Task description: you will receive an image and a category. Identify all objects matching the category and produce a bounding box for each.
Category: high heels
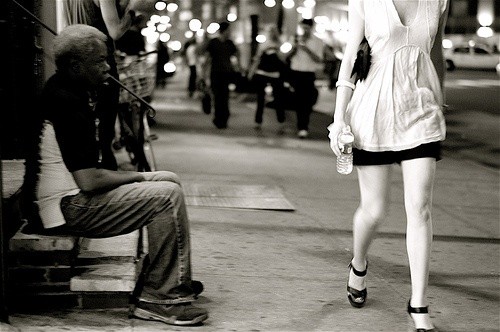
[347,257,368,308]
[408,299,435,332]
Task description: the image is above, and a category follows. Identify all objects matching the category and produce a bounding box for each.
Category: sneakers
[132,280,203,300]
[133,300,207,325]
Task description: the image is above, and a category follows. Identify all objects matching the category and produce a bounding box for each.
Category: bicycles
[116,51,158,173]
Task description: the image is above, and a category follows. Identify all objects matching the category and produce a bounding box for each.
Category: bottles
[337,125,354,175]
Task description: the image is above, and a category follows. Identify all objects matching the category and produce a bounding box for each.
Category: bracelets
[336,80,355,90]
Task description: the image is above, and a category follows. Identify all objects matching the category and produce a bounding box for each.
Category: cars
[443,42,500,72]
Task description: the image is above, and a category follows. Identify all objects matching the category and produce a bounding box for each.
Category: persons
[181,19,242,130]
[20,23,210,325]
[247,19,335,138]
[113,10,151,139]
[63,0,139,171]
[328,0,450,332]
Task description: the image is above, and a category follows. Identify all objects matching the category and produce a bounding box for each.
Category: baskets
[118,58,156,104]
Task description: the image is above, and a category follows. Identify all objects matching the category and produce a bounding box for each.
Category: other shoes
[254,122,263,132]
[278,123,286,133]
[301,131,308,138]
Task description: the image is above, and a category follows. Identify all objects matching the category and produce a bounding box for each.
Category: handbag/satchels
[202,93,211,113]
[350,37,371,81]
